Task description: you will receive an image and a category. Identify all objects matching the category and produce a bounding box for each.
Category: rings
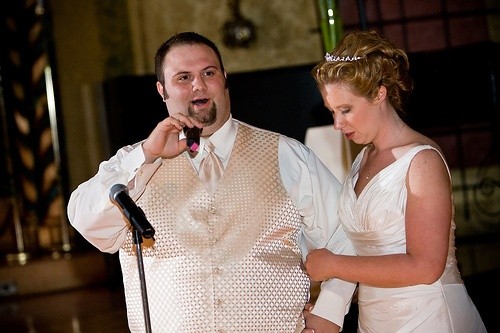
[309,306,314,311]
[312,329,315,333]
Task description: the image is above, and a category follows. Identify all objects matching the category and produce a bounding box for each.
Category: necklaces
[366,123,405,179]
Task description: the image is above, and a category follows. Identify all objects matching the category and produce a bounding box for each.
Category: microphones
[182,105,202,155]
[109,184,156,239]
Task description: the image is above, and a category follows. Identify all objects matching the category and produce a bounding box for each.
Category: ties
[196,139,227,197]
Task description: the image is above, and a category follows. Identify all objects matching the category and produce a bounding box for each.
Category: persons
[67,31,359,333]
[303,30,487,333]
